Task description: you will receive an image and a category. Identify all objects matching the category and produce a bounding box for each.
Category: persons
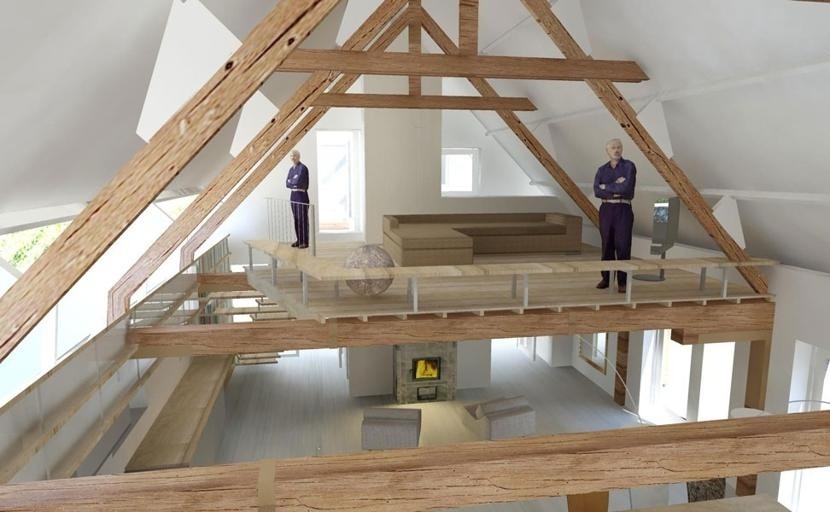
[285,148,310,249]
[592,138,637,293]
